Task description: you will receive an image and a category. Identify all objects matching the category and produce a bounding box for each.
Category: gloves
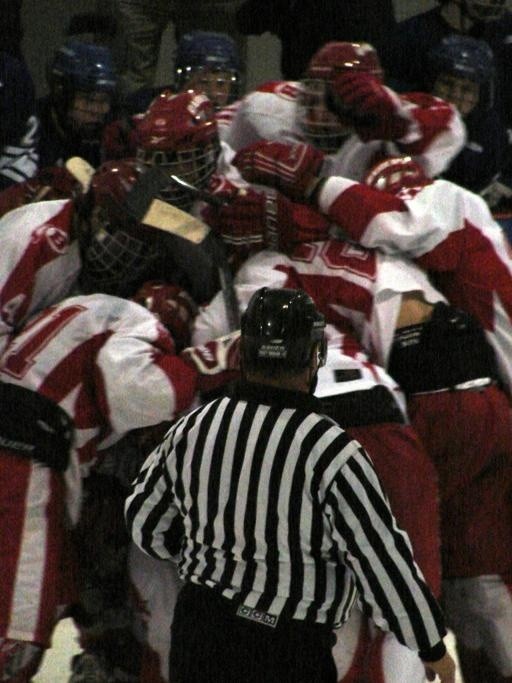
[182,330,244,394]
[321,70,417,145]
[201,186,332,254]
[229,135,327,202]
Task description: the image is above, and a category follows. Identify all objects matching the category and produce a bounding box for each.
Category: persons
[4,38,512,682]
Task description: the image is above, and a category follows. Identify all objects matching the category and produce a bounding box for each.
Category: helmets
[79,157,165,286]
[422,30,498,132]
[361,154,432,198]
[127,276,199,349]
[292,40,386,157]
[172,29,245,112]
[240,287,327,368]
[128,91,223,211]
[39,40,120,155]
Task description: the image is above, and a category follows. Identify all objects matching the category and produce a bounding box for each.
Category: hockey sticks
[169,172,222,243]
[138,198,240,332]
